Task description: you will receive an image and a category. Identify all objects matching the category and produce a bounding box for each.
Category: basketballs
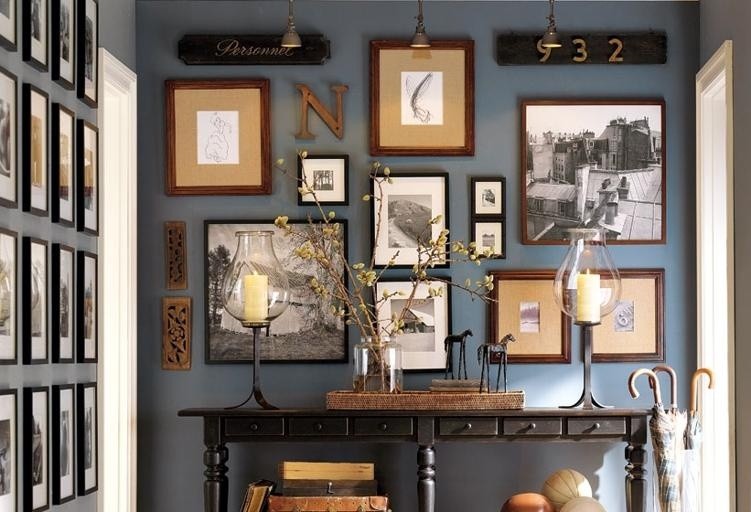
[542,468,593,511]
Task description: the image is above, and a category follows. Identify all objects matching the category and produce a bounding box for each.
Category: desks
[177,407,669,512]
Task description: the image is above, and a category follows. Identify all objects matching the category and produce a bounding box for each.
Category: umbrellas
[684,368,715,512]
[629,368,681,512]
[649,365,688,512]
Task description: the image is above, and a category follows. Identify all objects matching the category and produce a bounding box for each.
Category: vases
[352,336,404,393]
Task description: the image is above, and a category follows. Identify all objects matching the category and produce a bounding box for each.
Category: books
[240,461,378,512]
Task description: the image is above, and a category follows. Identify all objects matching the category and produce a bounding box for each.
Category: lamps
[409,1,431,47]
[541,0,563,48]
[282,0,304,48]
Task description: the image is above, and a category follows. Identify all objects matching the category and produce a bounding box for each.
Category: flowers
[270,147,500,394]
